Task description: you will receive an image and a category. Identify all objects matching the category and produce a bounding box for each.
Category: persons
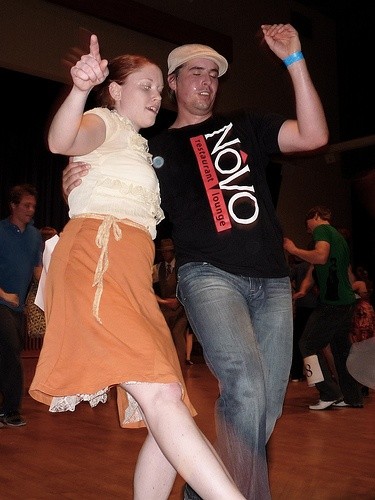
[44,33,250,500]
[0,180,374,426]
[61,22,328,500]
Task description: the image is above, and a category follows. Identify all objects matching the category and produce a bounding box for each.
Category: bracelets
[283,50,304,66]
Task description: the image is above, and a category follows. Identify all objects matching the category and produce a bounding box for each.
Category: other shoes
[309,397,343,410]
[333,401,362,407]
[185,360,194,366]
[0,414,27,428]
[184,483,203,500]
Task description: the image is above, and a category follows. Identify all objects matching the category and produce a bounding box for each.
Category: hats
[167,44,228,78]
[157,239,174,250]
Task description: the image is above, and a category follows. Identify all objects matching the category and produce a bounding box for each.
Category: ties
[167,265,171,279]
